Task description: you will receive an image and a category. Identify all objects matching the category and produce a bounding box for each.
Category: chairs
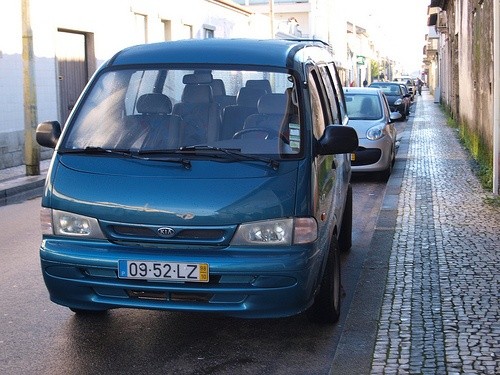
[112,93,183,149]
[173,85,222,147]
[221,88,268,140]
[391,87,396,91]
[360,97,373,113]
[240,93,287,139]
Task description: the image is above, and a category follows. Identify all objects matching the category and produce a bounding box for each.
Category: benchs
[198,80,272,109]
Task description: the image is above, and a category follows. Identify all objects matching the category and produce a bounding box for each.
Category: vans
[36,38,359,326]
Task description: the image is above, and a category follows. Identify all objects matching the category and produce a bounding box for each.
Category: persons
[417,81,423,95]
[363,79,367,87]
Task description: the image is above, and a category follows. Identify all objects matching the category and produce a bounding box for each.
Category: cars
[341,87,403,183]
[368,82,411,122]
[390,77,420,116]
